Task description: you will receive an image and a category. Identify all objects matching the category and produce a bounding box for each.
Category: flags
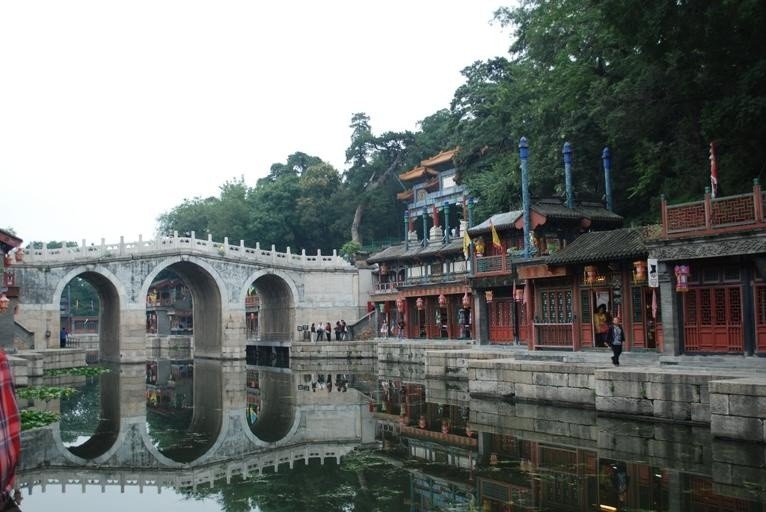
[491,224,501,249]
[709,142,718,193]
[463,229,471,261]
[652,288,657,319]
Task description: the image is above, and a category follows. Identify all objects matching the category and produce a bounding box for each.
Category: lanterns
[4,254,12,267]
[399,412,472,438]
[15,247,24,262]
[396,298,403,313]
[634,264,646,281]
[437,294,447,309]
[416,298,424,311]
[0,294,10,312]
[516,289,524,303]
[674,264,690,292]
[461,290,470,309]
[485,291,493,304]
[586,270,596,284]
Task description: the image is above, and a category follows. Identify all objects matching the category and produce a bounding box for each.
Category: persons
[381,379,407,400]
[605,317,625,367]
[448,224,453,237]
[397,316,405,339]
[381,319,389,339]
[60,328,68,348]
[611,462,629,504]
[311,320,348,343]
[594,304,611,348]
[312,374,348,392]
